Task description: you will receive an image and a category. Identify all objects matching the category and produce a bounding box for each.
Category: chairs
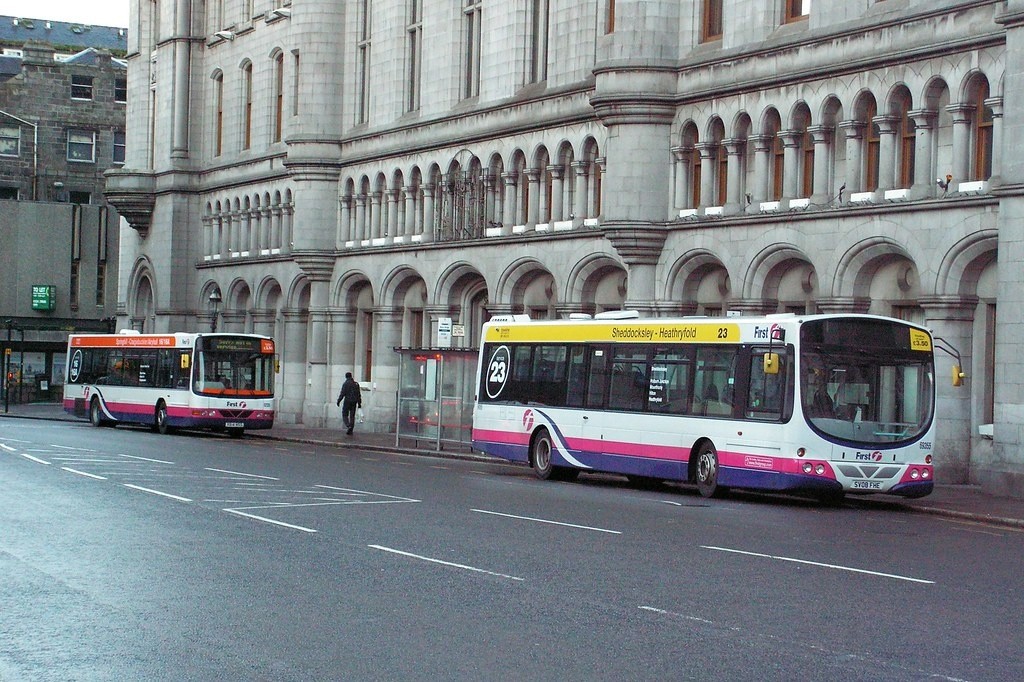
[814,390,838,419]
[695,384,734,417]
[514,358,643,403]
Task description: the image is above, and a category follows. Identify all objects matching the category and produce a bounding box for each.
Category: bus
[471,310,968,498]
[63,329,281,435]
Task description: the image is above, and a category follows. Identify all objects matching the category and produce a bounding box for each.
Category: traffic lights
[8,372,13,382]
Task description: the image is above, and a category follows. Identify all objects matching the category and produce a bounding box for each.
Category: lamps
[744,192,751,204]
[838,183,846,201]
[937,174,952,194]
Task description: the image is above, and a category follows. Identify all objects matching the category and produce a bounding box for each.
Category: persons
[337,372,362,435]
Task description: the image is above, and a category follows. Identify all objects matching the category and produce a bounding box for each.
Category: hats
[345,372,352,378]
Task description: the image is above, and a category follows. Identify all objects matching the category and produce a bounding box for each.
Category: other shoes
[346,426,353,435]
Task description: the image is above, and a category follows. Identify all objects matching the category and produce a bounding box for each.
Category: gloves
[337,399,340,407]
[358,404,362,408]
